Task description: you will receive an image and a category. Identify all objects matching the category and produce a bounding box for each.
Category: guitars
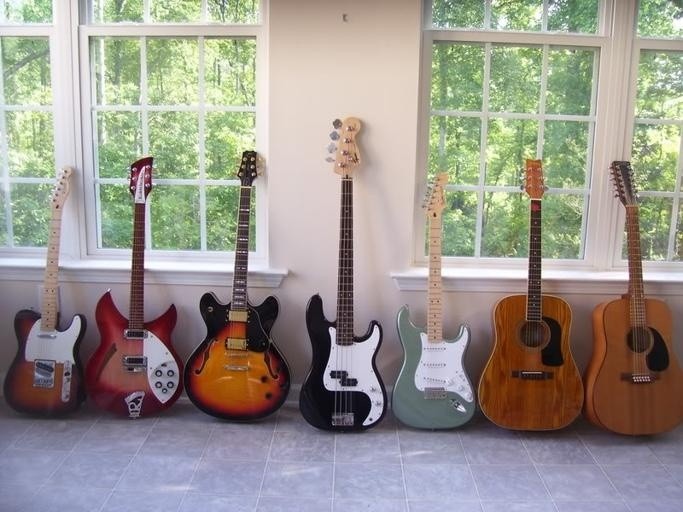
[4,167,87,418]
[391,170,477,431]
[299,118,387,432]
[478,159,585,432]
[86,158,184,419]
[183,150,292,422]
[583,161,683,437]
